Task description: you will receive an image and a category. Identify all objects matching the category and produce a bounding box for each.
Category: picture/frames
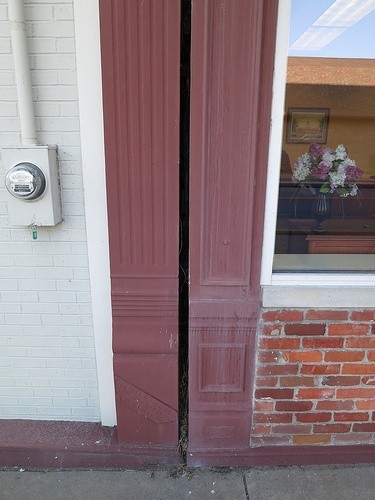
[285,106,331,145]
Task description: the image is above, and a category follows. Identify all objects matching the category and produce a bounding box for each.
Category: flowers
[289,143,365,198]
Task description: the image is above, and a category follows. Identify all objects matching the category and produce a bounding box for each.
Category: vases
[311,193,331,233]
